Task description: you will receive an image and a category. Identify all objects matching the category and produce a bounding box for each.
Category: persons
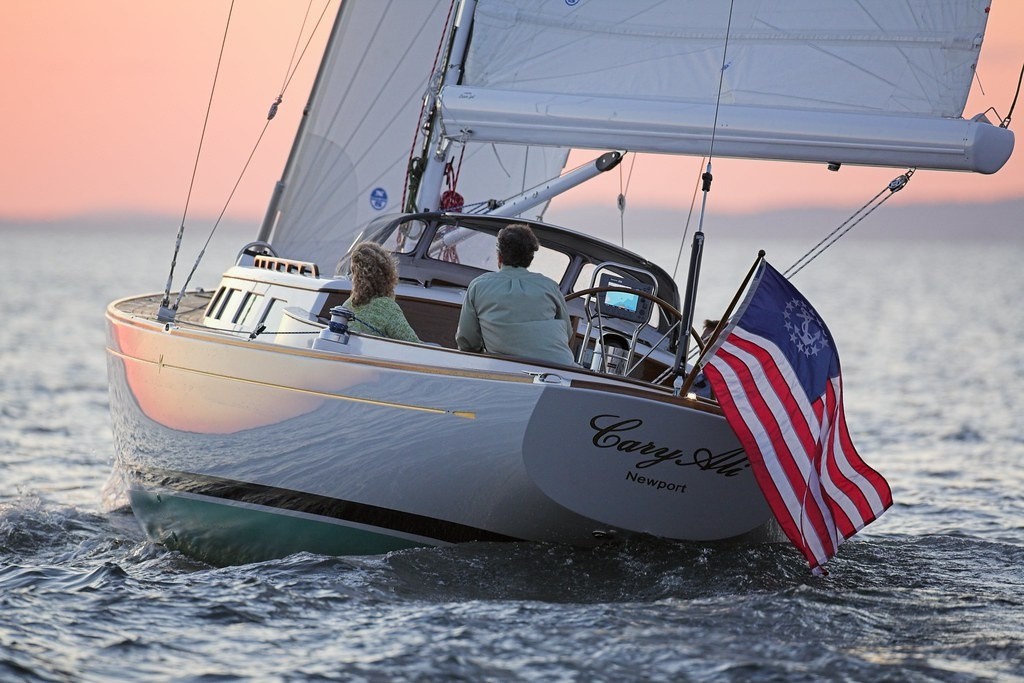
[456,224,577,366]
[342,242,423,347]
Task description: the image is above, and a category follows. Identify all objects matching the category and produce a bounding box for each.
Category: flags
[699,257,894,568]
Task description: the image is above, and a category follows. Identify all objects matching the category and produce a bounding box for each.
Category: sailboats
[106,0,1024,568]
